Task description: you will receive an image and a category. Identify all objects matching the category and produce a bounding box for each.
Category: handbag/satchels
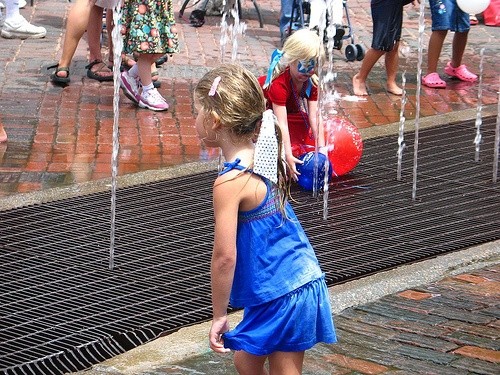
[482,0,500,27]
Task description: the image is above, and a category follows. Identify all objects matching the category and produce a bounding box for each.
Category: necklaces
[290,79,315,139]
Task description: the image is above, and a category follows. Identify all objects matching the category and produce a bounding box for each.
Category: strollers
[280,0,366,62]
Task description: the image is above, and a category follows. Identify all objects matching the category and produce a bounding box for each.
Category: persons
[195,0,352,49]
[0,0,48,40]
[422,1,478,88]
[0,121,8,149]
[193,68,337,375]
[352,0,414,97]
[47,0,181,111]
[251,28,329,182]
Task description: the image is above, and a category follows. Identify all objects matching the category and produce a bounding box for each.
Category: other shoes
[134,52,167,66]
[334,26,345,41]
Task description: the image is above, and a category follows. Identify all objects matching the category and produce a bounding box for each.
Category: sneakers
[1,15,46,39]
[121,71,138,103]
[0,0,27,8]
[139,88,169,110]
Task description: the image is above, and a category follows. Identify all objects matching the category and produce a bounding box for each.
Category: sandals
[421,72,446,88]
[152,71,161,87]
[85,59,113,81]
[47,64,70,83]
[108,56,132,72]
[445,62,478,82]
